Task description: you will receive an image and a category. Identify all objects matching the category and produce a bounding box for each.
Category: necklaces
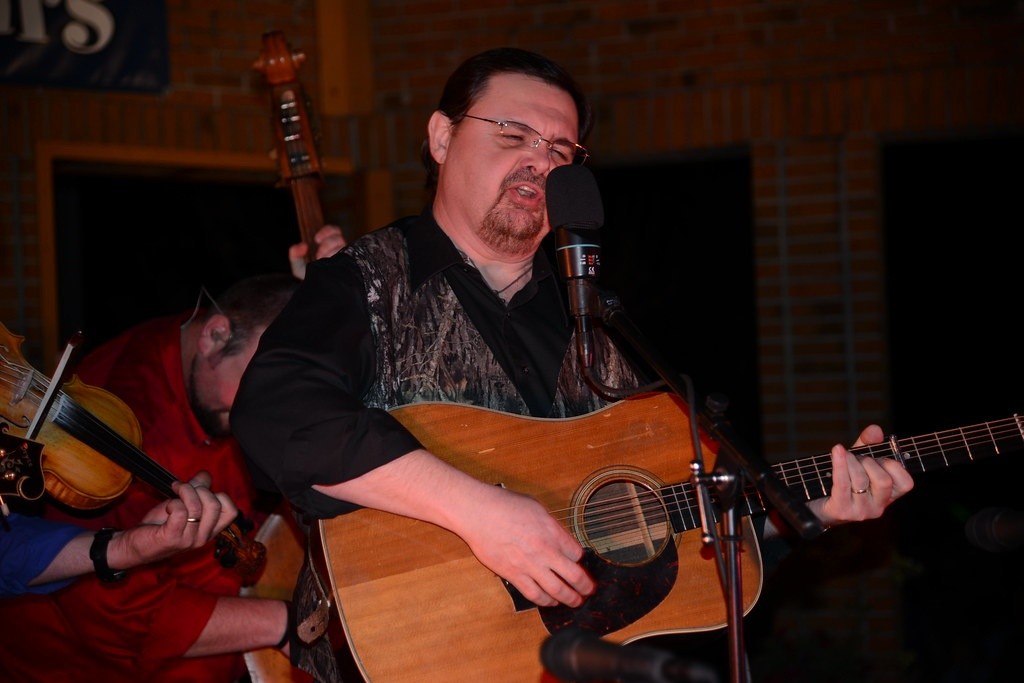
[459,250,532,304]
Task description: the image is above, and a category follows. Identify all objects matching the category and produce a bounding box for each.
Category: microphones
[541,627,719,683]
[965,507,1024,553]
[545,163,604,372]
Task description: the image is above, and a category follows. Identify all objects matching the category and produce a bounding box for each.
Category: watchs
[89,527,128,583]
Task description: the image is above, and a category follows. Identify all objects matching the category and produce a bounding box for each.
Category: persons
[0,469,239,598]
[229,48,916,683]
[0,224,350,683]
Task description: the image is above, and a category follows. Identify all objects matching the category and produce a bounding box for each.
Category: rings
[852,485,871,494]
[188,518,201,523]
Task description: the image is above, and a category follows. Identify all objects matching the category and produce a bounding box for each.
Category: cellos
[231,25,362,683]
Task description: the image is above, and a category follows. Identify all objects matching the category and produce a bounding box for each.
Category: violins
[0,320,268,569]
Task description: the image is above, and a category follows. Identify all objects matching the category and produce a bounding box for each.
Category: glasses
[462,113,595,168]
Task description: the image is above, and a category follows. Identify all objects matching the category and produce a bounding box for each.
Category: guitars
[307,375,1024,683]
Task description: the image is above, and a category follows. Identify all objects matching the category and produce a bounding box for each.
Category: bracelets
[275,599,294,650]
